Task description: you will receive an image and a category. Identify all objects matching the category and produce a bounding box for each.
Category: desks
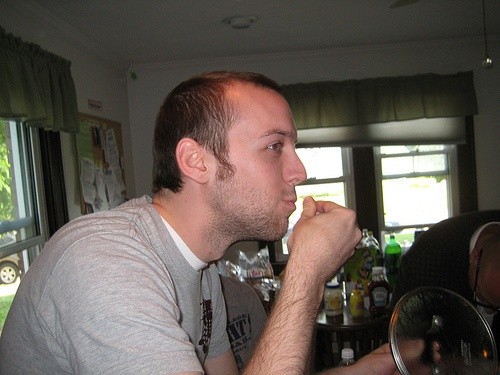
[315,300,389,369]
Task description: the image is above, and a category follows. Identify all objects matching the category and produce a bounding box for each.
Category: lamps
[222,15,258,29]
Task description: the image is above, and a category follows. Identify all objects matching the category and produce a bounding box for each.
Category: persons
[0,71,440,375]
[393,208,500,366]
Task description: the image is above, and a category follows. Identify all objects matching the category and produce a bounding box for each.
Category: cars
[0,255,20,285]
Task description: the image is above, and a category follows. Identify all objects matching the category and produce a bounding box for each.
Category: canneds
[350,287,364,318]
[324,282,344,317]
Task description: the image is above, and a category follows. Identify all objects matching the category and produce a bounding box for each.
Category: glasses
[471,248,500,312]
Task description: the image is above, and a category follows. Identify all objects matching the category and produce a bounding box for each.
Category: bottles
[344,227,402,318]
[338,347,355,368]
[324,282,344,318]
